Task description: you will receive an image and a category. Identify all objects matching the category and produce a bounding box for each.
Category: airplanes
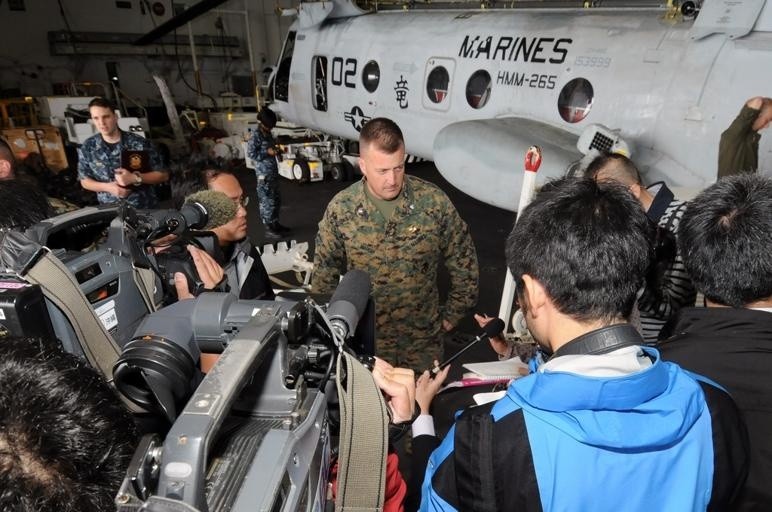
[255,1,771,215]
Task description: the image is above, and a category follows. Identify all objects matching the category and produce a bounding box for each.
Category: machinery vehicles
[0,95,71,182]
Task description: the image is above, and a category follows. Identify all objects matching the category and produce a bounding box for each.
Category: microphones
[427,318,506,376]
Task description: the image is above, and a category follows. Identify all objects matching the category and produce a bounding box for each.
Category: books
[462,356,528,381]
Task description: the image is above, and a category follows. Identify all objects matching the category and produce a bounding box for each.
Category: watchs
[134,172,142,183]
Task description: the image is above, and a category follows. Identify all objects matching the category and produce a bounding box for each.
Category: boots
[265,221,292,238]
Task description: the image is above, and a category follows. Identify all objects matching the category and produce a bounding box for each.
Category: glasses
[233,195,250,208]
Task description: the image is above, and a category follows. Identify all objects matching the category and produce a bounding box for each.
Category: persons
[418,178,752,512]
[585,152,690,348]
[655,174,772,512]
[77,97,171,208]
[1,179,225,373]
[416,359,451,479]
[0,334,416,512]
[246,107,289,236]
[0,139,17,181]
[165,156,276,302]
[312,118,479,376]
[473,312,552,393]
